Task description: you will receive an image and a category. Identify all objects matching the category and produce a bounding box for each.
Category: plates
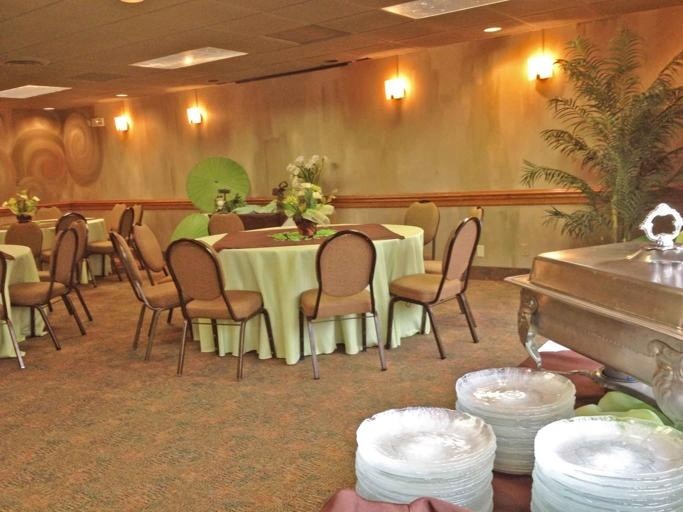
[527,416,681,511]
[453,369,576,476]
[352,405,495,512]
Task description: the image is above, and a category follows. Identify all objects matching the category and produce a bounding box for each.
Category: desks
[324,339,608,512]
[502,240,682,426]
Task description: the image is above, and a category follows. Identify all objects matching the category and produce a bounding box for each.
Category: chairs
[404,202,441,260]
[297,228,386,380]
[1,211,97,370]
[132,222,175,325]
[208,213,247,233]
[108,230,193,365]
[87,203,144,287]
[385,206,482,360]
[166,239,277,380]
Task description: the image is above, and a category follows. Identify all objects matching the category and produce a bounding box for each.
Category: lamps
[114,116,130,133]
[384,77,404,100]
[527,54,553,80]
[187,107,202,124]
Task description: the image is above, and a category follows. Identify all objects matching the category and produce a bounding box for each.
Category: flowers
[281,154,337,227]
[2,185,39,217]
